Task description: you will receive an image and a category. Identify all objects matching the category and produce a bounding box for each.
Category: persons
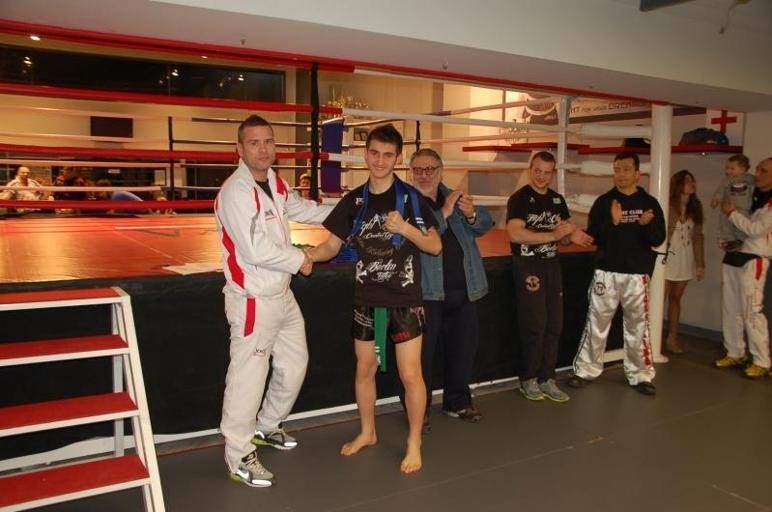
[60,166,89,215]
[395,144,499,438]
[206,110,338,492]
[565,149,668,398]
[92,178,151,214]
[0,164,48,217]
[710,152,756,253]
[660,168,708,356]
[303,121,446,477]
[43,172,65,197]
[294,171,312,200]
[711,156,772,381]
[503,149,597,405]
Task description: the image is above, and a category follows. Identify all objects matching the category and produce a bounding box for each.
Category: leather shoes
[669,340,685,356]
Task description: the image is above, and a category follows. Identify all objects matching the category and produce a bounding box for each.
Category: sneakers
[725,241,744,252]
[520,377,544,400]
[636,383,655,397]
[253,424,297,450]
[746,363,769,378]
[228,454,276,488]
[541,378,570,403]
[570,374,592,386]
[403,409,432,434]
[444,408,485,423]
[714,354,746,367]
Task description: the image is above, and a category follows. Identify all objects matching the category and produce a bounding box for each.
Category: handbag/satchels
[679,128,728,145]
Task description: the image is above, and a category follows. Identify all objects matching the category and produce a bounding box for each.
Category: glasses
[412,167,440,176]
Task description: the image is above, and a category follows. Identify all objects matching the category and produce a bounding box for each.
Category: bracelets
[465,211,478,220]
